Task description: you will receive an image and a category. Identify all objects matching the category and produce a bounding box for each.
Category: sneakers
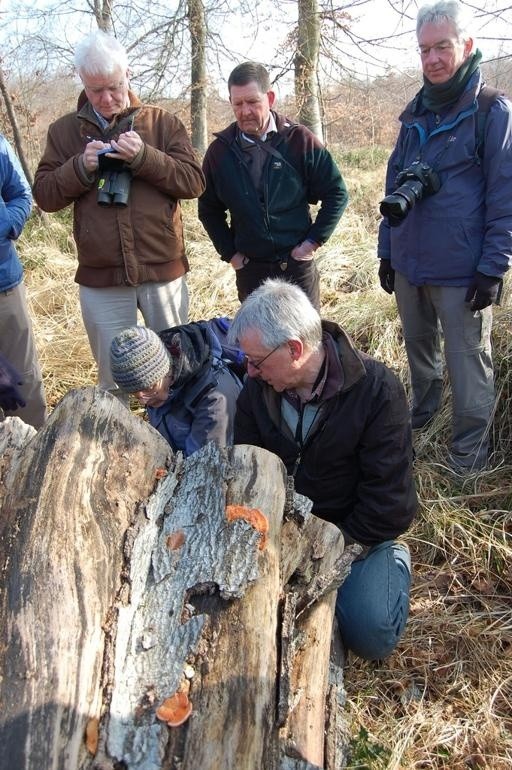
[393,540,411,569]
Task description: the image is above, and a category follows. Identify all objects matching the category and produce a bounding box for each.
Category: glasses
[241,347,279,371]
[416,44,455,56]
[131,385,162,401]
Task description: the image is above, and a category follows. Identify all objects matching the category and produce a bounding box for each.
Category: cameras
[379,162,441,227]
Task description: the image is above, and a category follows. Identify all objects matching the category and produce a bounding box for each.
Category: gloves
[377,260,396,294]
[0,360,26,415]
[465,271,501,312]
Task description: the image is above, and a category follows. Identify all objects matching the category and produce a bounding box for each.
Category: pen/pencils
[86,136,97,142]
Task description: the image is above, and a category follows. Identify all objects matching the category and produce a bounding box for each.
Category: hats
[110,326,174,391]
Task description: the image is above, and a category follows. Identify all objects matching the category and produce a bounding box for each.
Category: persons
[0,354,26,413]
[197,62,349,318]
[376,0,512,475]
[232,276,418,661]
[110,316,243,459]
[31,29,207,413]
[0,128,48,428]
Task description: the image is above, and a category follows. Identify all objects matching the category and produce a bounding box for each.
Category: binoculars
[97,169,132,209]
